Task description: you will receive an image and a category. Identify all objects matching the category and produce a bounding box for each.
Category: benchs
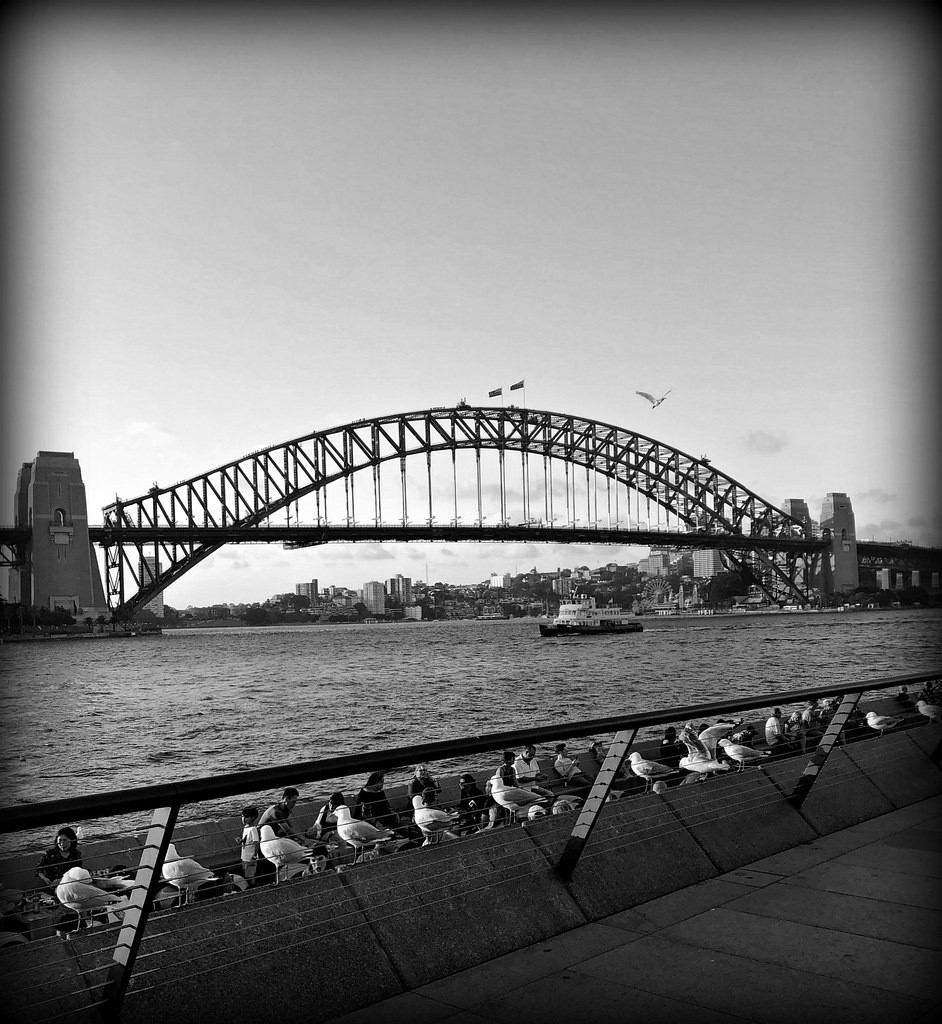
[0,692,929,951]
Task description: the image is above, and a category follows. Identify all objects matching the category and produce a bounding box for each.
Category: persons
[765,696,845,752]
[512,744,554,807]
[469,781,504,822]
[895,679,942,709]
[699,720,759,749]
[235,806,259,886]
[408,764,442,816]
[314,793,346,855]
[555,743,595,787]
[449,774,485,814]
[660,726,688,766]
[257,788,328,858]
[36,827,83,895]
[354,772,399,833]
[496,751,519,788]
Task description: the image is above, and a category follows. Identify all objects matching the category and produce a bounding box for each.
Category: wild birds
[625,752,679,794]
[260,825,313,887]
[57,867,122,934]
[162,844,218,907]
[329,805,394,865]
[914,700,942,726]
[636,390,669,410]
[717,737,771,773]
[863,712,904,738]
[412,795,460,846]
[487,775,548,826]
[679,722,735,780]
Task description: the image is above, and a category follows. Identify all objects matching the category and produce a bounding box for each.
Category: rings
[55,879,57,881]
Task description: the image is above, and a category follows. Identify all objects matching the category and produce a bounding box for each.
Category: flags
[510,380,523,391]
[488,388,502,398]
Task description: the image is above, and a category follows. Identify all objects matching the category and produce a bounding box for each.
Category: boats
[539,593,644,637]
[478,613,510,620]
